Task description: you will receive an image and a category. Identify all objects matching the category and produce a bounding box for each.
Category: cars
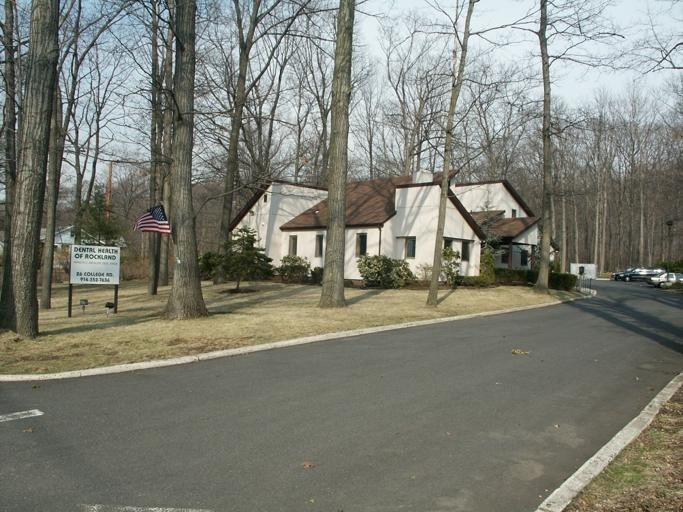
[609,266,665,282]
[646,271,682,289]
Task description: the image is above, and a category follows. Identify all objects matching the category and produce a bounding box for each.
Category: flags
[131,203,171,235]
